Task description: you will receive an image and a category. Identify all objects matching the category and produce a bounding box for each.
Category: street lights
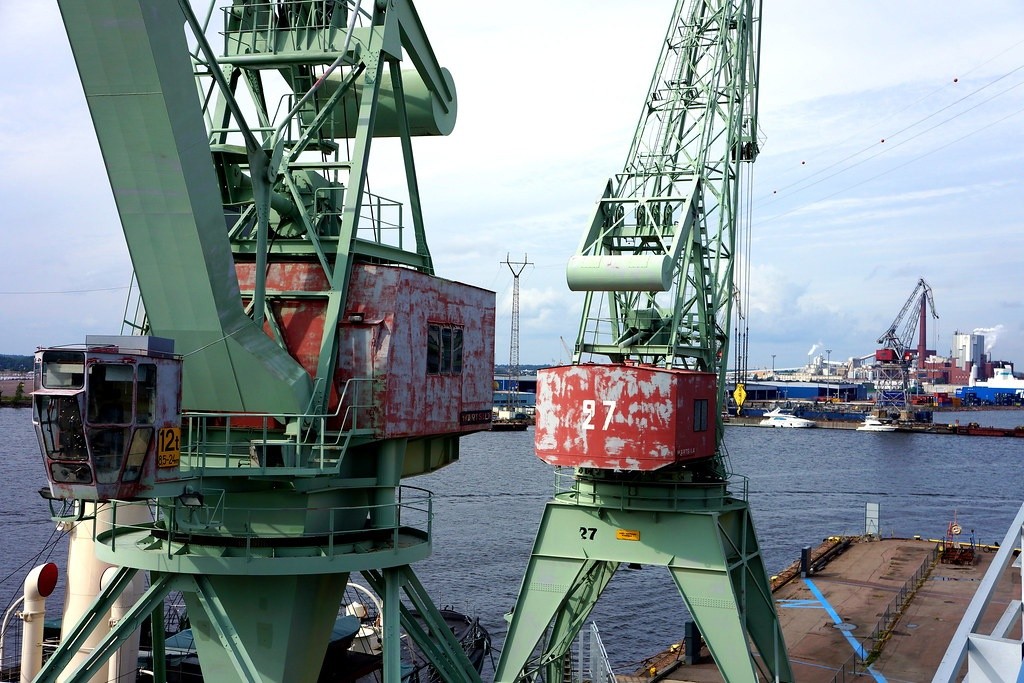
[783,390,788,403]
[825,349,832,402]
[776,390,781,402]
[771,354,777,376]
[834,393,838,398]
[844,392,849,402]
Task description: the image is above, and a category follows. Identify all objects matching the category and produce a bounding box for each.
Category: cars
[721,411,736,418]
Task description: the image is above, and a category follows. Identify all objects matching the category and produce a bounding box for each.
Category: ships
[605,498,1024,683]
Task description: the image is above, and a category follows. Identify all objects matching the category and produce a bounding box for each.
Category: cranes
[559,335,574,366]
[492,0,802,681]
[20,3,505,681]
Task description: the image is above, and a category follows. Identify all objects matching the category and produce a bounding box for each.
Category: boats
[856,415,900,431]
[490,407,528,432]
[0,576,491,683]
[759,403,817,429]
